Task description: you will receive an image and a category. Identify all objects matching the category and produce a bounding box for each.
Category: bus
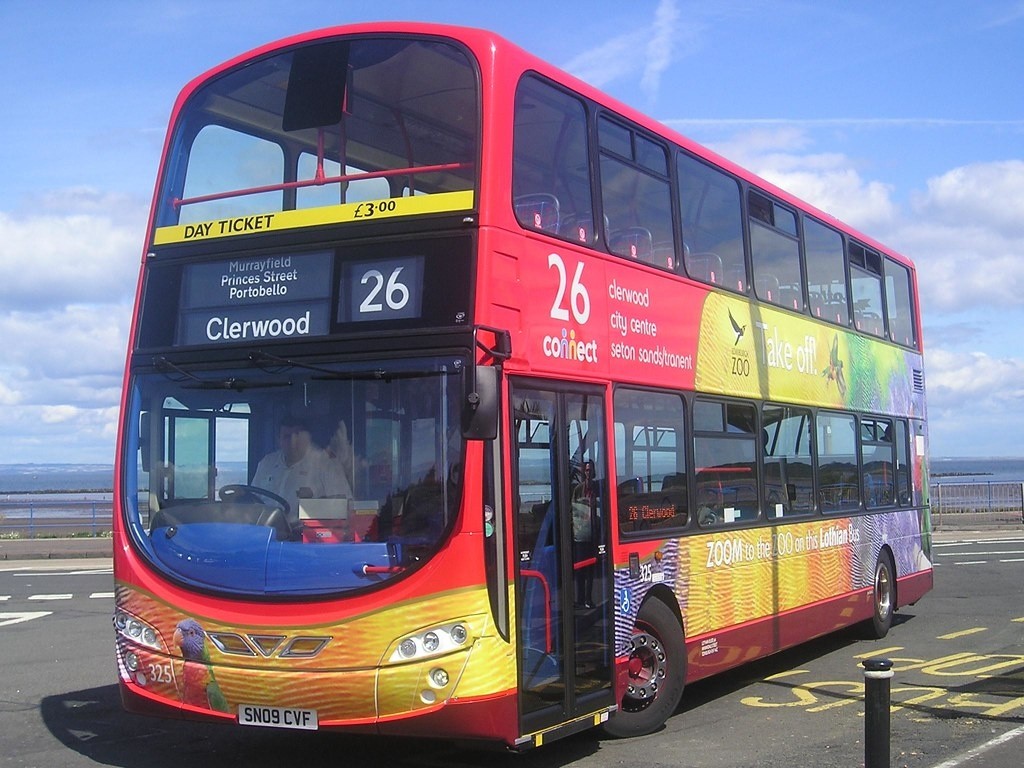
[111,21,934,751]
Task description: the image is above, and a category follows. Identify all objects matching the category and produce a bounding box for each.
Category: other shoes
[571,602,584,609]
[585,602,596,608]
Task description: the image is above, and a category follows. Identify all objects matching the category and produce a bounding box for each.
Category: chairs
[570,457,911,537]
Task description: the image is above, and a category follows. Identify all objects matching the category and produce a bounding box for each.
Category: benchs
[514,191,912,346]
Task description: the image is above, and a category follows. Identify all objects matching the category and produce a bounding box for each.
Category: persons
[251,417,354,531]
[573,461,596,611]
